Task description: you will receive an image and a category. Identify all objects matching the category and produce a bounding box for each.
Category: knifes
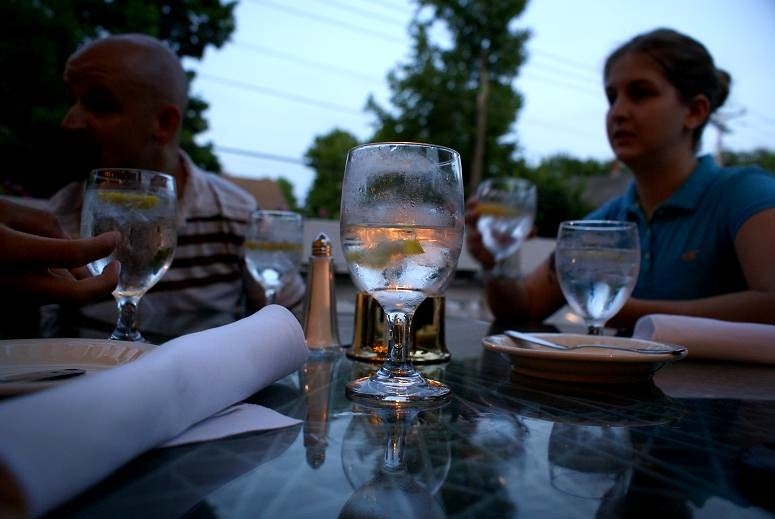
[503,327,686,354]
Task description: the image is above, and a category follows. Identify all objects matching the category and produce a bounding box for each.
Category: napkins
[160,402,304,447]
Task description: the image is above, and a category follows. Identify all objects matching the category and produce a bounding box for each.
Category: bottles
[301,231,343,350]
[297,356,336,467]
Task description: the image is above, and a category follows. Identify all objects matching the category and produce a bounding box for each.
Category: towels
[653,364,775,402]
[633,312,775,363]
[0,302,307,518]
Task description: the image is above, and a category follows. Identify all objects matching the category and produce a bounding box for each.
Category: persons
[465,29,775,328]
[0,34,306,344]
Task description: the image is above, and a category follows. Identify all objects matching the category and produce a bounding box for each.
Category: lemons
[98,191,158,209]
[475,204,518,215]
[348,239,422,261]
[245,241,303,249]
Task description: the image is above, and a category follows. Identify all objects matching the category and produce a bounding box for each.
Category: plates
[481,333,688,381]
[0,337,159,396]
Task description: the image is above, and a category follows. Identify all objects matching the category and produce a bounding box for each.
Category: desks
[0,309,775,519]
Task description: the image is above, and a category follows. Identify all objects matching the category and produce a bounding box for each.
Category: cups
[549,422,632,499]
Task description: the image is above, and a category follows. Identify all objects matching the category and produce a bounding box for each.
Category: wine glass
[555,219,641,336]
[474,176,537,282]
[81,168,177,344]
[339,141,465,404]
[338,397,452,519]
[244,209,304,307]
